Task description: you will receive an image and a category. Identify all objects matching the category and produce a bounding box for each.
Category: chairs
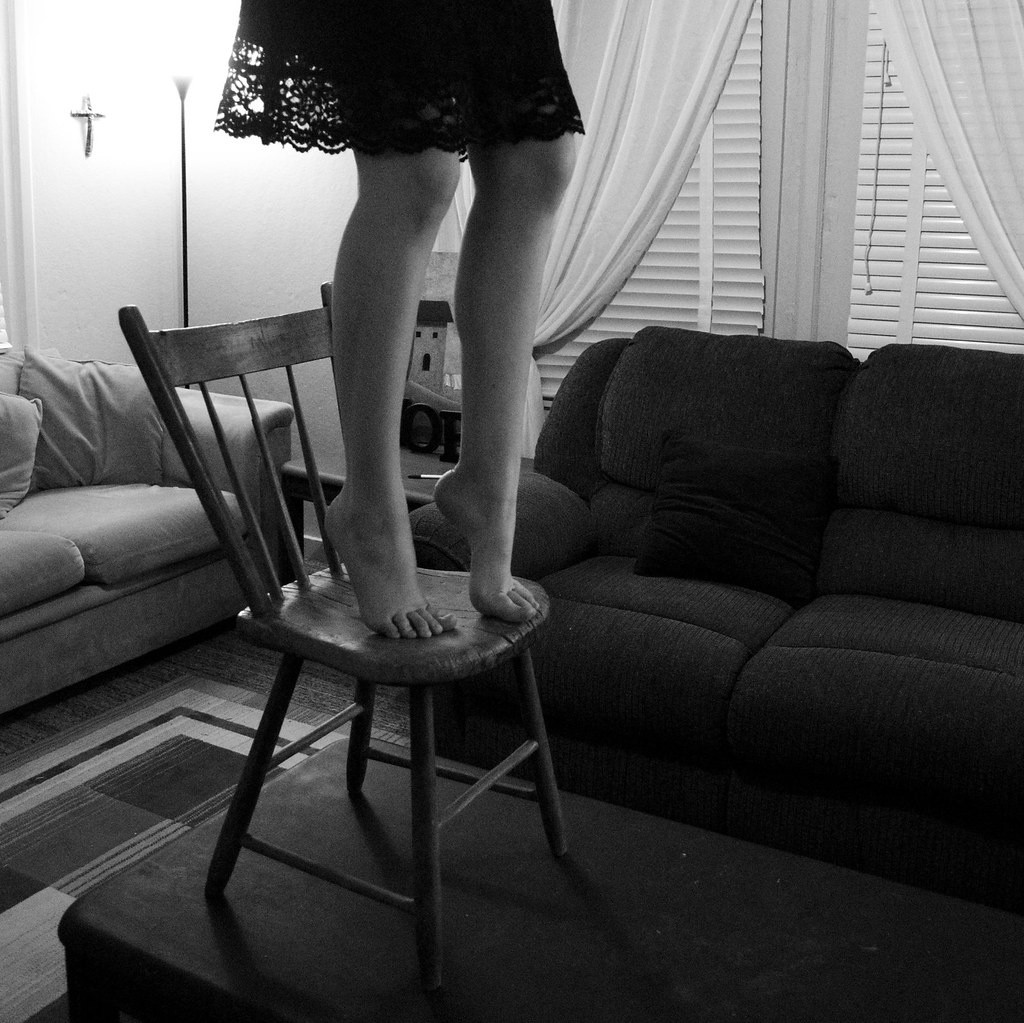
[119,280,568,992]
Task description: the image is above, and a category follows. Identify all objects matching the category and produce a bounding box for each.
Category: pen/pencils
[408,474,446,480]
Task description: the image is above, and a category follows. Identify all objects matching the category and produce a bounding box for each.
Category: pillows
[631,430,837,608]
[17,346,165,486]
[0,392,43,519]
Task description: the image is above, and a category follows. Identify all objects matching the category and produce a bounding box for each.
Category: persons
[214,0,586,636]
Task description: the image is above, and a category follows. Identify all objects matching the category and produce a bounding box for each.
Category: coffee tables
[57,738,1024,1023]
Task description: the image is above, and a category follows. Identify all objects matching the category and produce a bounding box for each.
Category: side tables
[277,446,533,586]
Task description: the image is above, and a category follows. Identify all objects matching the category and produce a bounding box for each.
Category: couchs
[406,326,1023,916]
[0,346,296,730]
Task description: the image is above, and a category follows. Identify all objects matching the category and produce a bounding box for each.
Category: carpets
[0,673,410,1023]
